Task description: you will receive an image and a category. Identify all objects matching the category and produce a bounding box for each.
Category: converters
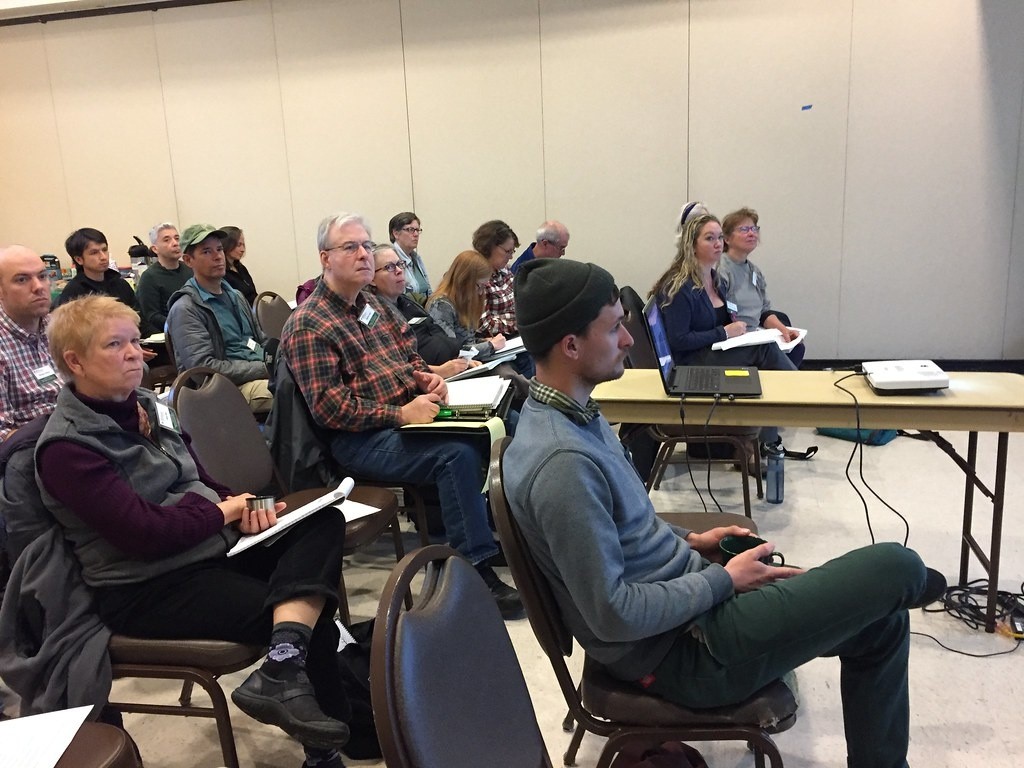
[1010,617,1024,641]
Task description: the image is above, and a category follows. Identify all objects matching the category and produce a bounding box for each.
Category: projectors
[860,358,951,396]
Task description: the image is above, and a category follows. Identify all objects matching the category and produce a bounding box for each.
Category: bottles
[138,263,148,284]
[62,264,77,278]
[764,441,785,504]
[121,272,134,278]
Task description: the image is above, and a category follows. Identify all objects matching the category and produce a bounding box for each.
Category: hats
[512,258,613,349]
[179,223,228,253]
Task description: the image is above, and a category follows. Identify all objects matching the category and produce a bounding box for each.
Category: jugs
[128,236,158,279]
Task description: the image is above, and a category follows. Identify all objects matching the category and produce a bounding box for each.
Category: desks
[590,369,1024,633]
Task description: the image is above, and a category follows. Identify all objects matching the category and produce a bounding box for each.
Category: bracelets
[724,327,728,339]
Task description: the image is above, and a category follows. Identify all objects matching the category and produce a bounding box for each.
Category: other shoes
[914,567,947,610]
[489,578,527,620]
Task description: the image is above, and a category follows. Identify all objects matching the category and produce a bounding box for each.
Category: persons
[0,243,73,446]
[500,257,946,768]
[282,212,569,613]
[651,208,805,459]
[32,294,382,767]
[60,222,276,421]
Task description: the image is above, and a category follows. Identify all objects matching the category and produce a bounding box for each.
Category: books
[482,336,526,361]
[437,379,504,408]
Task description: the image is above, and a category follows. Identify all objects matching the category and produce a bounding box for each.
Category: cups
[245,496,276,518]
[720,535,785,567]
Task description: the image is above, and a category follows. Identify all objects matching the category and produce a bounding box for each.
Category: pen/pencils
[413,394,448,408]
[487,328,494,338]
[731,314,736,322]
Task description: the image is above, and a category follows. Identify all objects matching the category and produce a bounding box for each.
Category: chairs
[37,286,799,768]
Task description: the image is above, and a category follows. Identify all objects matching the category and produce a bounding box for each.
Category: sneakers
[232,669,351,749]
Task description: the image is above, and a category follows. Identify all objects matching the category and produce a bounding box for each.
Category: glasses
[732,226,760,233]
[495,244,516,256]
[401,227,422,234]
[375,260,406,272]
[542,239,568,251]
[325,240,377,254]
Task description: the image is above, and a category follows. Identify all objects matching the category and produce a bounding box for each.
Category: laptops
[642,295,763,398]
